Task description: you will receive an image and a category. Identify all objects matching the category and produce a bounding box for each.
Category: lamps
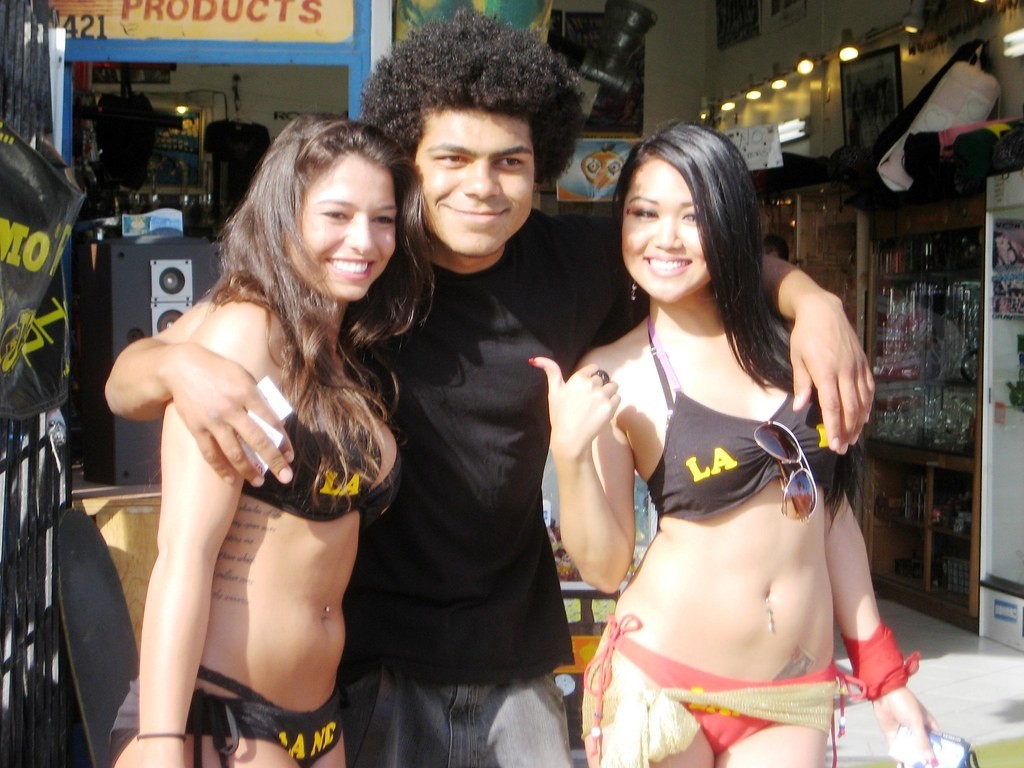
[697,0,925,120]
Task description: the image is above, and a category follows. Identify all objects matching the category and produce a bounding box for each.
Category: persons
[106,4,877,768]
[529,120,941,768]
[763,235,788,262]
[108,114,433,768]
[993,232,1024,313]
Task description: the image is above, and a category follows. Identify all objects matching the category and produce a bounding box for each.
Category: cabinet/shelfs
[755,185,866,334]
[862,193,993,639]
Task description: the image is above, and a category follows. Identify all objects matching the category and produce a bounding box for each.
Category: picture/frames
[838,44,903,158]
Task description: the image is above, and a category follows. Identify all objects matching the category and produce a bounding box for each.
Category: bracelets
[137,733,187,742]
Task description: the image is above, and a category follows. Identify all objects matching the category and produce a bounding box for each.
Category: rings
[591,369,609,386]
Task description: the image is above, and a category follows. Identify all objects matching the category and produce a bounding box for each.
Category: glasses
[754,420,817,523]
